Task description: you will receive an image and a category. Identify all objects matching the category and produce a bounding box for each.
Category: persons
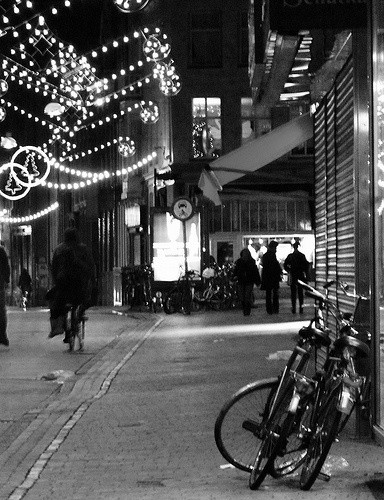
[230,249,262,316]
[45,226,97,343]
[0,244,12,347]
[192,105,215,158]
[18,267,34,302]
[283,241,310,316]
[202,252,231,270]
[260,241,283,315]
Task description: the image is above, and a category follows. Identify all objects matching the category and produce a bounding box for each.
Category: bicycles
[165,264,254,315]
[65,304,85,352]
[214,279,372,490]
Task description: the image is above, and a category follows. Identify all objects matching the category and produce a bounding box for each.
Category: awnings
[195,106,312,207]
